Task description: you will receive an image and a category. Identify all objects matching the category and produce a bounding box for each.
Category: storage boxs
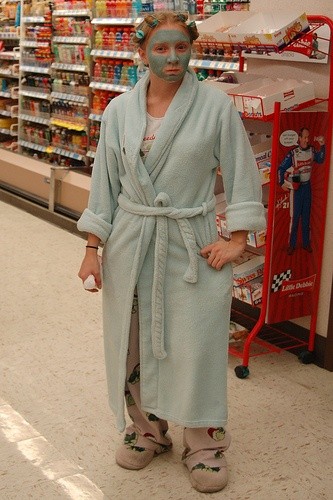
[190,9,318,380]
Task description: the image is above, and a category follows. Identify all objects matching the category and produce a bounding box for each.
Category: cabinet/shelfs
[1,0,252,249]
[181,15,333,378]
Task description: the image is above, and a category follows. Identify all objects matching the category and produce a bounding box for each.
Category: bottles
[0,0,248,175]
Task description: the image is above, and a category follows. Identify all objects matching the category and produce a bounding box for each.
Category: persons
[78,10,267,493]
[277,127,326,255]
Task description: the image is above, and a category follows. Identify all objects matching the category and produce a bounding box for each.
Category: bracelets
[86,246,98,250]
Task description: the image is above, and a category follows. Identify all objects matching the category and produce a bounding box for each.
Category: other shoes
[116,444,154,470]
[287,247,294,255]
[189,466,228,493]
[303,246,312,253]
